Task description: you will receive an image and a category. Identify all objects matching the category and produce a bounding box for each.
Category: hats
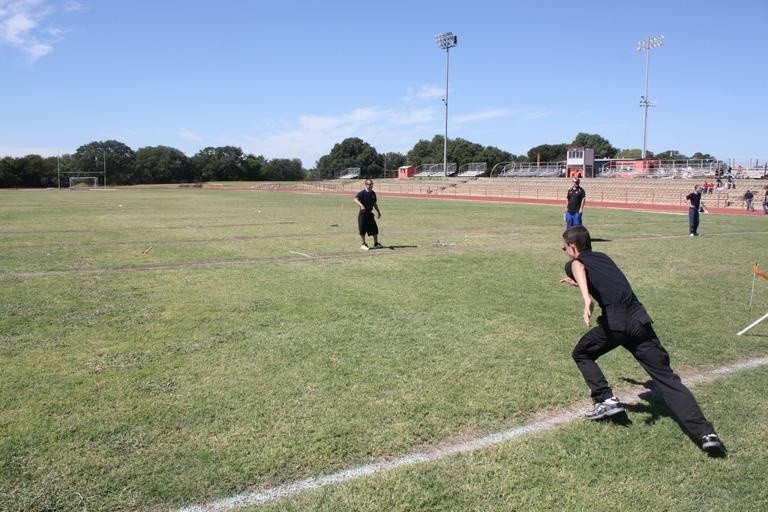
[573,178,580,183]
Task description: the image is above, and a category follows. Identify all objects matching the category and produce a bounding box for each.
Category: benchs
[296,173,767,214]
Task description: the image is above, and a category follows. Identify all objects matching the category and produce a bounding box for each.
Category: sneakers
[361,243,382,250]
[701,433,727,457]
[585,397,625,420]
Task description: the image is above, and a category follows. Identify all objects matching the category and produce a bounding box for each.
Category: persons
[714,176,721,188]
[561,178,586,251]
[706,182,713,193]
[557,227,728,456]
[702,181,707,193]
[353,179,381,249]
[726,174,735,188]
[762,185,767,213]
[685,183,703,236]
[743,190,755,212]
